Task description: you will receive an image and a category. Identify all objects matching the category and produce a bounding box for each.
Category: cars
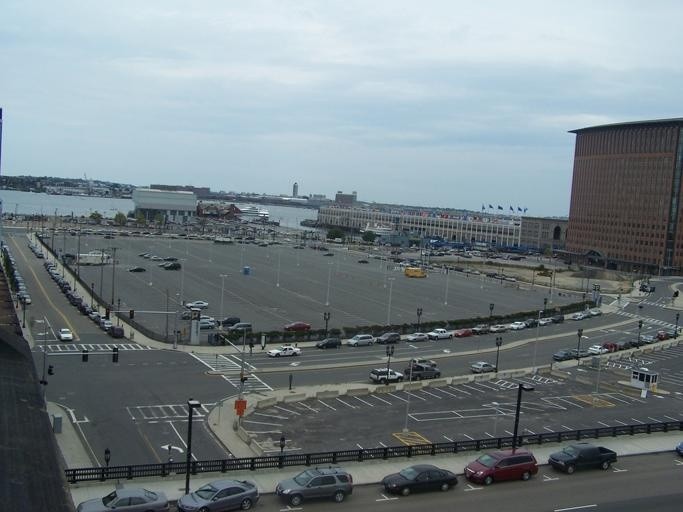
[178,298,314,331]
[175,232,281,246]
[41,260,127,341]
[54,248,62,252]
[266,344,302,358]
[376,244,543,286]
[568,306,604,322]
[1,237,31,308]
[314,310,564,350]
[549,327,675,364]
[359,259,368,265]
[128,251,183,273]
[27,241,46,260]
[640,282,657,293]
[44,224,166,241]
[470,360,498,374]
[677,438,683,458]
[283,237,334,258]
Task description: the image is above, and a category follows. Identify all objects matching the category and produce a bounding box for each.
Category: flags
[481,204,527,213]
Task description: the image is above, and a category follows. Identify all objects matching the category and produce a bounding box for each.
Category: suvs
[369,357,442,382]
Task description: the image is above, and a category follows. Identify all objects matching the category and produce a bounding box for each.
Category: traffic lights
[47,364,55,379]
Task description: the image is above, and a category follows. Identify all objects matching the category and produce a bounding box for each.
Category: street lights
[413,305,425,332]
[673,311,681,339]
[509,381,534,455]
[490,333,504,371]
[636,319,644,349]
[542,296,549,316]
[183,395,207,496]
[380,340,395,379]
[487,301,496,327]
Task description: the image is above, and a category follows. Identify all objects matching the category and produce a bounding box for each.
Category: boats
[240,205,282,226]
[300,218,322,228]
